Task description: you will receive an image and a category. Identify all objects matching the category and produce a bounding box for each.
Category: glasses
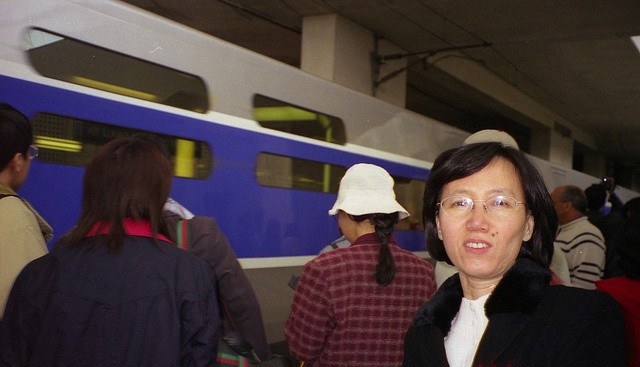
[27,145,40,161]
[437,195,526,218]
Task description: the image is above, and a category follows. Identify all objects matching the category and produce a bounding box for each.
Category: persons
[584,184,627,280]
[401,140,633,367]
[549,184,608,292]
[594,195,640,367]
[158,195,269,365]
[1,102,55,320]
[601,175,625,213]
[1,134,223,366]
[435,129,574,292]
[282,163,437,367]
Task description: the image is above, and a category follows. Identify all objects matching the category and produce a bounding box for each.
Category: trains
[0,0,640,346]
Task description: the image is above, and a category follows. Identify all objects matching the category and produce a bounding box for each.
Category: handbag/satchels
[215,337,305,367]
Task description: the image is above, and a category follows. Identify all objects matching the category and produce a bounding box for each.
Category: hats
[328,163,410,221]
[463,129,520,150]
